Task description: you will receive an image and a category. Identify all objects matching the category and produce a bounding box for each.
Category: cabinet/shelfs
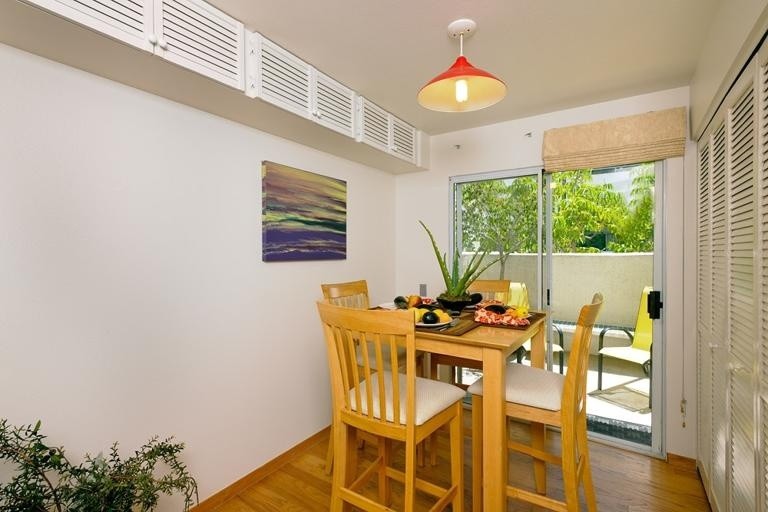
[689,45,767,512]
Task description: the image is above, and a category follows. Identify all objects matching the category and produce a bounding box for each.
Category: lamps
[416,20,508,115]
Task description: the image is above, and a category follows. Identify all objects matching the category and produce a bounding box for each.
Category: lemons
[408,307,451,323]
[518,308,528,318]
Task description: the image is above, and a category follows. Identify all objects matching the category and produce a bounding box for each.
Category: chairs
[318,281,651,512]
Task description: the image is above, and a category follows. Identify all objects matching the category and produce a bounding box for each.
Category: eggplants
[416,304,460,316]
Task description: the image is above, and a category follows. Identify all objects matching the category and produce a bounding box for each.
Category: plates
[416,320,452,327]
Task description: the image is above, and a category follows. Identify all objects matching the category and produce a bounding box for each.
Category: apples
[409,296,422,308]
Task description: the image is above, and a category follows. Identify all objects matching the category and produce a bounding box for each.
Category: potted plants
[419,220,517,314]
